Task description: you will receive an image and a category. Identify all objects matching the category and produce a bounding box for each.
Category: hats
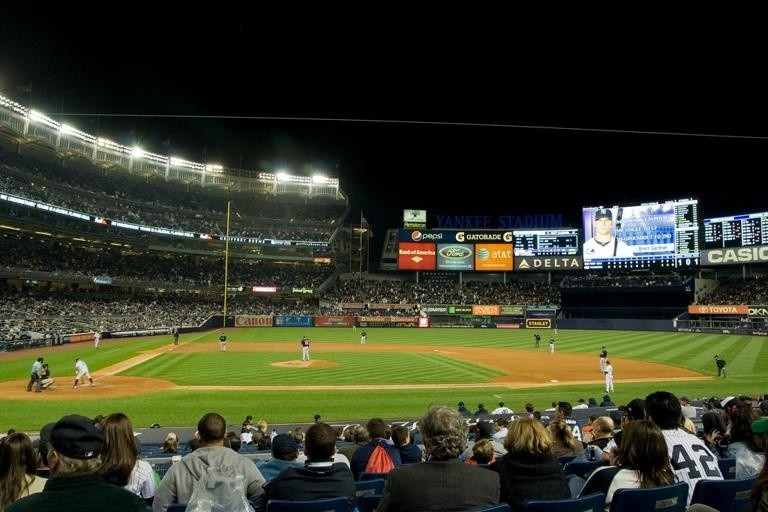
[719,395,743,410]
[617,397,647,421]
[49,414,104,460]
[272,433,302,453]
[474,420,494,438]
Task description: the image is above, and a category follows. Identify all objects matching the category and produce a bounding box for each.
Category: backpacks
[362,444,398,475]
[181,453,255,512]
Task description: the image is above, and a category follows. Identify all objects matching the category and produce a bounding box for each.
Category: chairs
[137,419,767,511]
